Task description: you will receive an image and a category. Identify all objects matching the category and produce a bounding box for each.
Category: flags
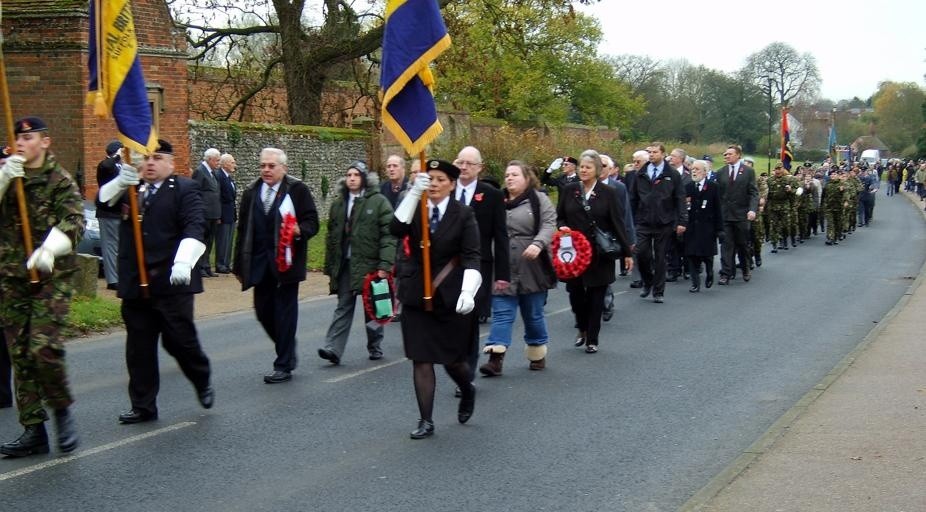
[828,119,837,154]
[85,0,162,156]
[378,0,451,159]
[781,106,793,175]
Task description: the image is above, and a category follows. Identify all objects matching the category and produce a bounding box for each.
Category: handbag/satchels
[593,226,624,260]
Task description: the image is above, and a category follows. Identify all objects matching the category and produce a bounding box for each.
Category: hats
[155,139,173,155]
[563,156,578,164]
[760,162,811,176]
[106,141,123,155]
[480,175,502,189]
[744,156,753,167]
[0,144,12,157]
[702,155,712,162]
[15,117,49,135]
[829,162,868,175]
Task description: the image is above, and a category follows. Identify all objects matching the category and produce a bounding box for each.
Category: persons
[233,147,319,383]
[318,160,398,365]
[886,157,926,200]
[94,139,215,423]
[0,147,12,409]
[541,145,767,353]
[0,117,87,457]
[383,147,559,440]
[216,155,238,273]
[95,141,126,290]
[759,157,883,253]
[191,148,221,277]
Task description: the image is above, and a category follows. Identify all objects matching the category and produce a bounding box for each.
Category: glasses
[602,164,609,168]
[456,159,481,167]
[633,159,647,164]
[258,161,285,170]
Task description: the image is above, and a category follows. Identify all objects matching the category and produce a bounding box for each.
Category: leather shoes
[196,376,214,408]
[603,292,614,321]
[618,269,664,303]
[119,407,157,422]
[665,258,762,292]
[410,418,435,439]
[200,269,237,277]
[318,348,340,364]
[264,339,297,383]
[574,332,599,353]
[369,351,382,359]
[455,385,475,424]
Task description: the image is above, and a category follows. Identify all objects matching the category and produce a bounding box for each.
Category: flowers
[402,234,411,258]
[474,192,484,202]
[548,229,592,279]
[274,210,297,274]
[362,272,401,325]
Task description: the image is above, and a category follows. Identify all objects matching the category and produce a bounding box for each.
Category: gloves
[0,155,28,199]
[26,227,74,274]
[455,268,483,316]
[100,162,141,208]
[169,238,208,287]
[393,171,431,225]
[546,157,563,174]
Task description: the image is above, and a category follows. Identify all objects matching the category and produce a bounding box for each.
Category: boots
[479,344,508,376]
[524,344,547,370]
[1,421,49,457]
[766,224,825,252]
[825,217,872,245]
[54,408,79,452]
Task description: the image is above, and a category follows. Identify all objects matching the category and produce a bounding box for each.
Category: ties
[460,189,465,203]
[652,168,657,182]
[696,183,700,191]
[730,166,734,182]
[264,188,274,215]
[429,208,440,234]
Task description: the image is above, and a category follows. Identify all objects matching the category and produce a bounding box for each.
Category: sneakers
[106,282,117,289]
